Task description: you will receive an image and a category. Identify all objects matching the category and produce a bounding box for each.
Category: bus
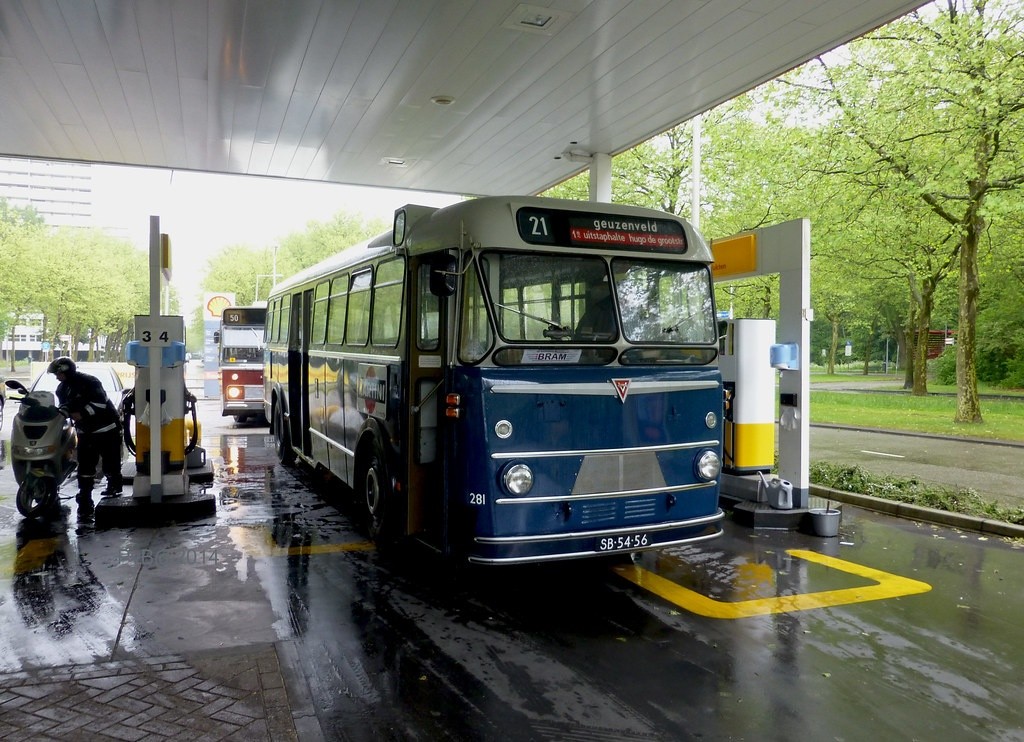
[213,302,270,423]
[263,195,725,569]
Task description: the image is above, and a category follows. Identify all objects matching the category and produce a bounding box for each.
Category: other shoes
[76,489,92,501]
[101,486,122,495]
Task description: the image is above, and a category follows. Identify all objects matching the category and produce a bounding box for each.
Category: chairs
[577,293,648,343]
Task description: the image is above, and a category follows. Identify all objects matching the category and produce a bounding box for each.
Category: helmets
[47,355,76,373]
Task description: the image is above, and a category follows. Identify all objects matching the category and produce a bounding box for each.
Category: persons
[46,356,124,507]
[571,284,652,360]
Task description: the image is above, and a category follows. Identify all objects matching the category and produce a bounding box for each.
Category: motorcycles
[6,380,79,519]
